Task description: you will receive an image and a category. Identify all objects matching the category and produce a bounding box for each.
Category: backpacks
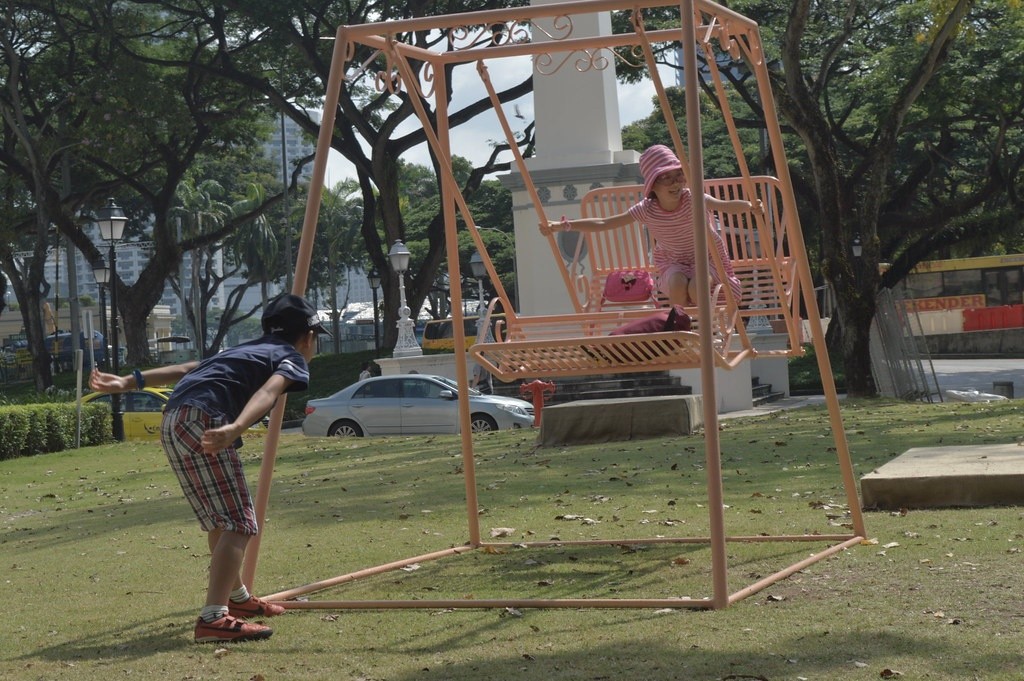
[602,269,654,301]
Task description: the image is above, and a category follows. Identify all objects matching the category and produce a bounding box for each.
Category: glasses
[655,173,684,185]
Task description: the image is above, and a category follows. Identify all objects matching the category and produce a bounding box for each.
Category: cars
[301,374,536,437]
[70,387,270,443]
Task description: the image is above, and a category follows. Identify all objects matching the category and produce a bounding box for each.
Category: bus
[878,254,1024,359]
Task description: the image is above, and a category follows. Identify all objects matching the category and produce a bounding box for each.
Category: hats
[261,294,333,337]
[639,144,682,197]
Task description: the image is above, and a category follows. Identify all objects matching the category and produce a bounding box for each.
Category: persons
[89,292,334,641]
[538,144,768,306]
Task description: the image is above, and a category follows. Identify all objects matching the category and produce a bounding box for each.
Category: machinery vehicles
[9,301,106,375]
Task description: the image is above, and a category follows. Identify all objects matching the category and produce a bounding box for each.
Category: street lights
[386,239,423,358]
[367,264,382,358]
[92,254,113,374]
[475,226,518,314]
[468,250,499,358]
[93,197,130,443]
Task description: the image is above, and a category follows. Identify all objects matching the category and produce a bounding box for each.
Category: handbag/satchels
[590,303,692,363]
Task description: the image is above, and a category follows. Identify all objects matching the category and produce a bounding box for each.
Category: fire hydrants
[518,379,554,428]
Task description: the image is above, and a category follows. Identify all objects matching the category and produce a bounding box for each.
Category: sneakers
[193,613,273,643]
[228,594,286,618]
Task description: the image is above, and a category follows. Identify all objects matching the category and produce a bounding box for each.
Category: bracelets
[561,214,571,233]
[130,368,147,391]
